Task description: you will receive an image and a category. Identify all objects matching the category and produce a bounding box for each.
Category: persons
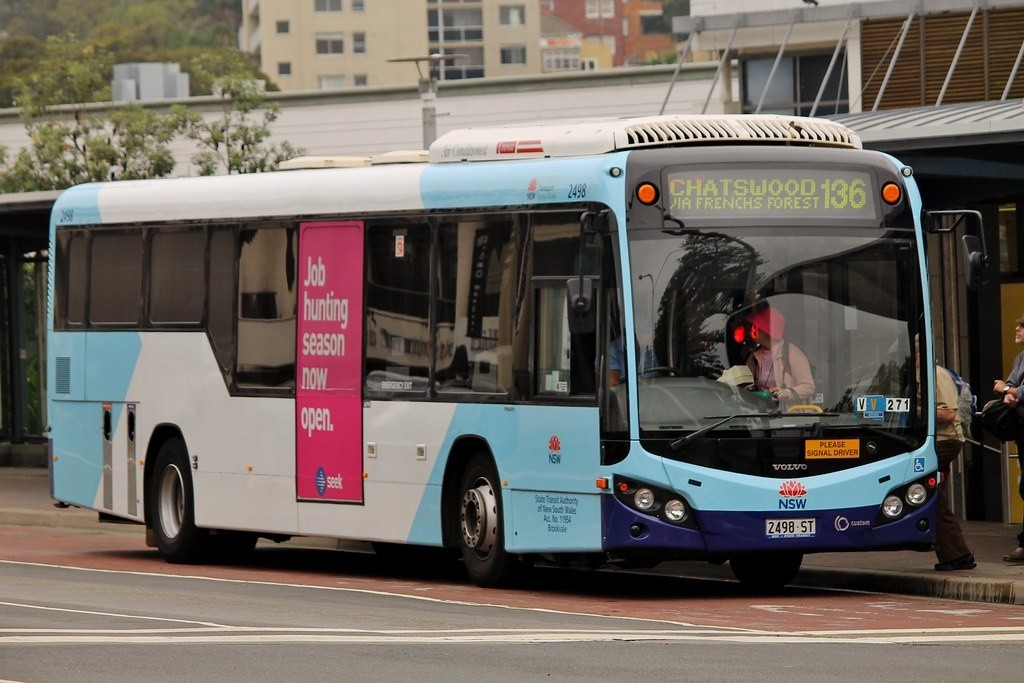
[993,315,1024,564]
[607,316,660,387]
[745,305,816,401]
[918,334,977,572]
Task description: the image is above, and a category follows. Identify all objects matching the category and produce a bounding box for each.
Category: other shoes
[934,552,976,570]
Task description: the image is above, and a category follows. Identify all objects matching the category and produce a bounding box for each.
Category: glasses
[1016,323,1024,328]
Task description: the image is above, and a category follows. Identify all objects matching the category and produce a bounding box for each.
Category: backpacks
[948,369,978,426]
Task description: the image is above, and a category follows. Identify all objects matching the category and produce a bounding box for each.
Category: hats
[1015,312,1024,323]
[744,307,786,340]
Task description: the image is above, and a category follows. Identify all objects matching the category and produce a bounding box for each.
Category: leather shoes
[1004,547,1024,561]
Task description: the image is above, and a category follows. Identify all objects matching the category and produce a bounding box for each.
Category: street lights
[384,54,469,152]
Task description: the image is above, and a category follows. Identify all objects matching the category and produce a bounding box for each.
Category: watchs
[1003,385,1011,395]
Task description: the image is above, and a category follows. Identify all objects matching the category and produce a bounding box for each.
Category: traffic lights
[724,298,781,410]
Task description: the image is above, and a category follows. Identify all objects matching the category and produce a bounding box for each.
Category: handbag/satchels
[981,380,1024,443]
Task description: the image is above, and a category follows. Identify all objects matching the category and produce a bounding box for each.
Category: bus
[45,112,994,588]
